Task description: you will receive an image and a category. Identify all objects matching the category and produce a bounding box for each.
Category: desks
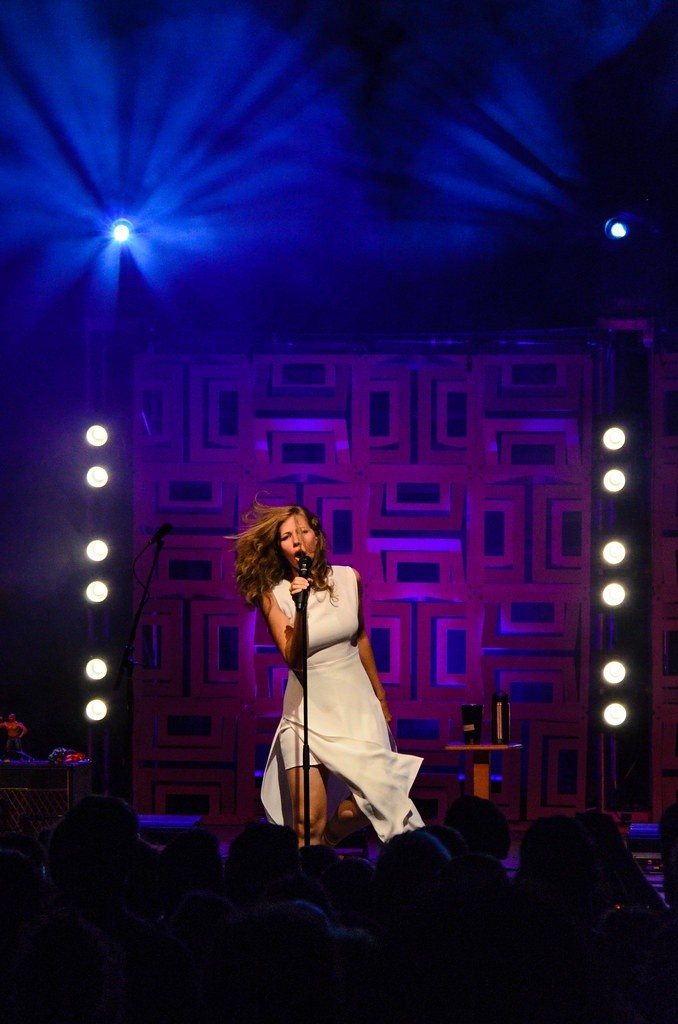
[446,743,523,801]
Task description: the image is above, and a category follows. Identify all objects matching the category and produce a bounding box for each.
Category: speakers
[0,759,93,840]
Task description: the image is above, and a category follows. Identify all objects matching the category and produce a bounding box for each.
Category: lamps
[592,649,648,733]
[598,532,639,611]
[593,416,641,499]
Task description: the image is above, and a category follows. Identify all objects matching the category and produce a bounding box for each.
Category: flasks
[491,690,511,745]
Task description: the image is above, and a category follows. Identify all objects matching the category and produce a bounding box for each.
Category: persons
[231,506,425,853]
[0,795,678,1024]
[0,714,27,752]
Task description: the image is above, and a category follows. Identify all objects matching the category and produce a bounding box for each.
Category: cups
[461,703,483,745]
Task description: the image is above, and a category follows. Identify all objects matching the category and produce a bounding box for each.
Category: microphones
[148,523,173,545]
[296,556,313,611]
[17,813,36,833]
